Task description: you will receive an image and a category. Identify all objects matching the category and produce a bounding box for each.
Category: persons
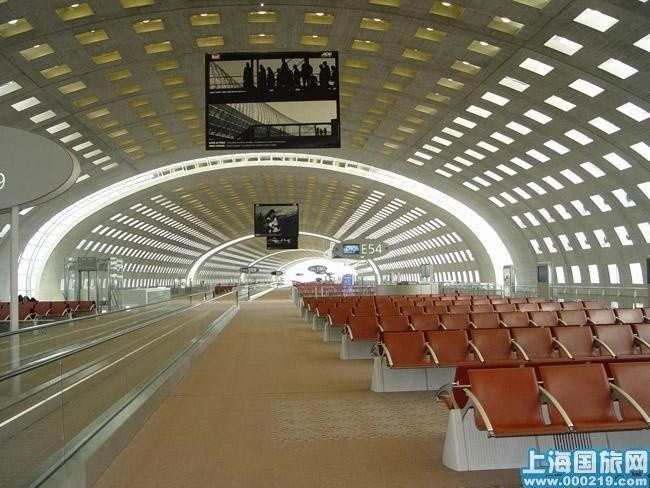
[30,298,35,302]
[243,57,338,90]
[18,295,23,302]
[316,128,327,136]
[23,296,29,302]
[263,209,281,234]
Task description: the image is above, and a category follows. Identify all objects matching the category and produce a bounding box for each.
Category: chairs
[213,285,236,293]
[289,278,649,471]
[0,299,97,325]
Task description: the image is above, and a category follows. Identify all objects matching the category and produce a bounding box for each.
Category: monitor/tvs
[343,244,360,254]
